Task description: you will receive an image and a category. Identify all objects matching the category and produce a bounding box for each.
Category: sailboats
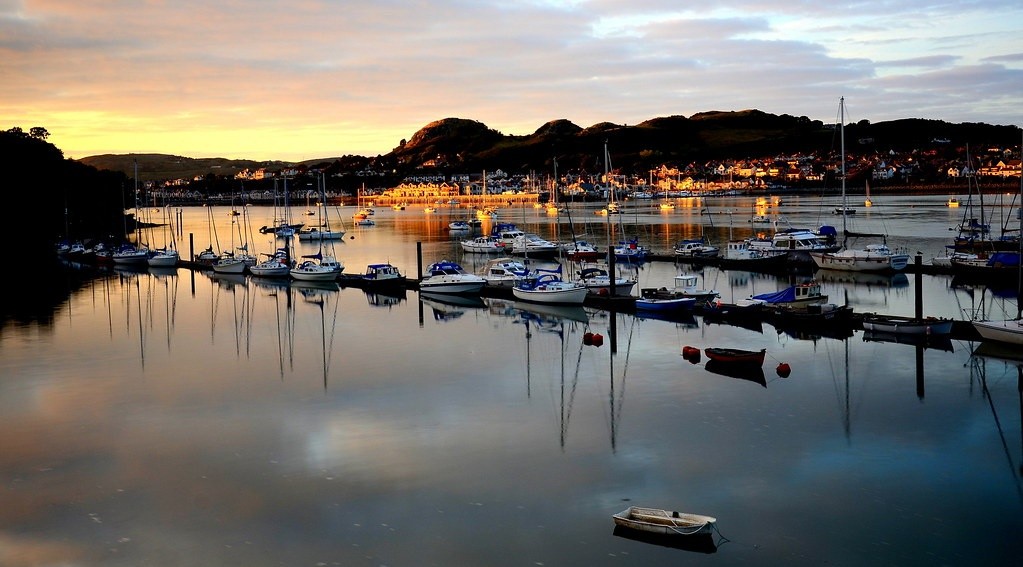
[809,95,910,271]
[932,143,1022,275]
[197,172,344,280]
[205,273,340,392]
[55,162,180,267]
[60,262,179,371]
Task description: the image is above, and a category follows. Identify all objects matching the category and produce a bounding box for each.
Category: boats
[863,331,955,354]
[705,348,766,365]
[361,285,407,312]
[261,224,346,239]
[420,293,488,324]
[352,183,374,226]
[392,204,405,210]
[513,302,589,445]
[724,95,838,316]
[613,506,717,537]
[963,298,1023,342]
[964,342,1023,367]
[419,144,720,313]
[362,255,407,284]
[705,361,768,388]
[863,317,953,335]
